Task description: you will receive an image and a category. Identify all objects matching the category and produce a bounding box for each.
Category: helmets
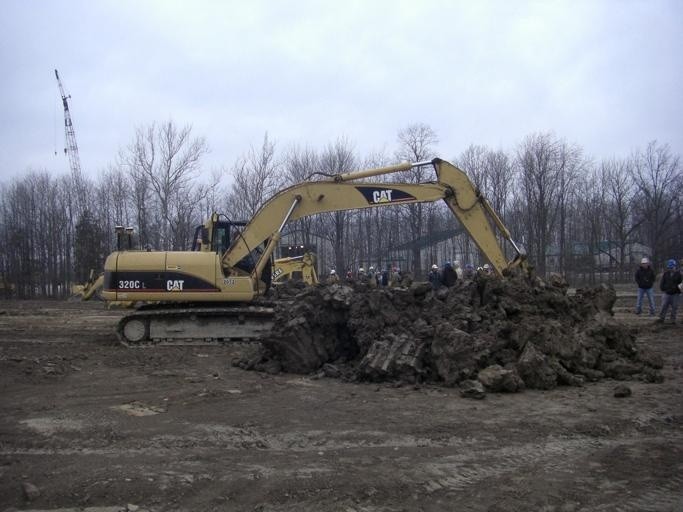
[640,257,650,265]
[664,258,678,269]
[329,264,402,276]
[431,260,491,271]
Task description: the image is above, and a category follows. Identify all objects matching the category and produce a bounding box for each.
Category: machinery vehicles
[191,211,322,290]
[67,272,106,303]
[84,157,532,350]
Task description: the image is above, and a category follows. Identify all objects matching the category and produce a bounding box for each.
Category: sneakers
[634,308,678,325]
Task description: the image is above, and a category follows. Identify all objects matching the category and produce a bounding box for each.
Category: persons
[635,258,656,315]
[657,259,683,324]
[328,259,493,291]
